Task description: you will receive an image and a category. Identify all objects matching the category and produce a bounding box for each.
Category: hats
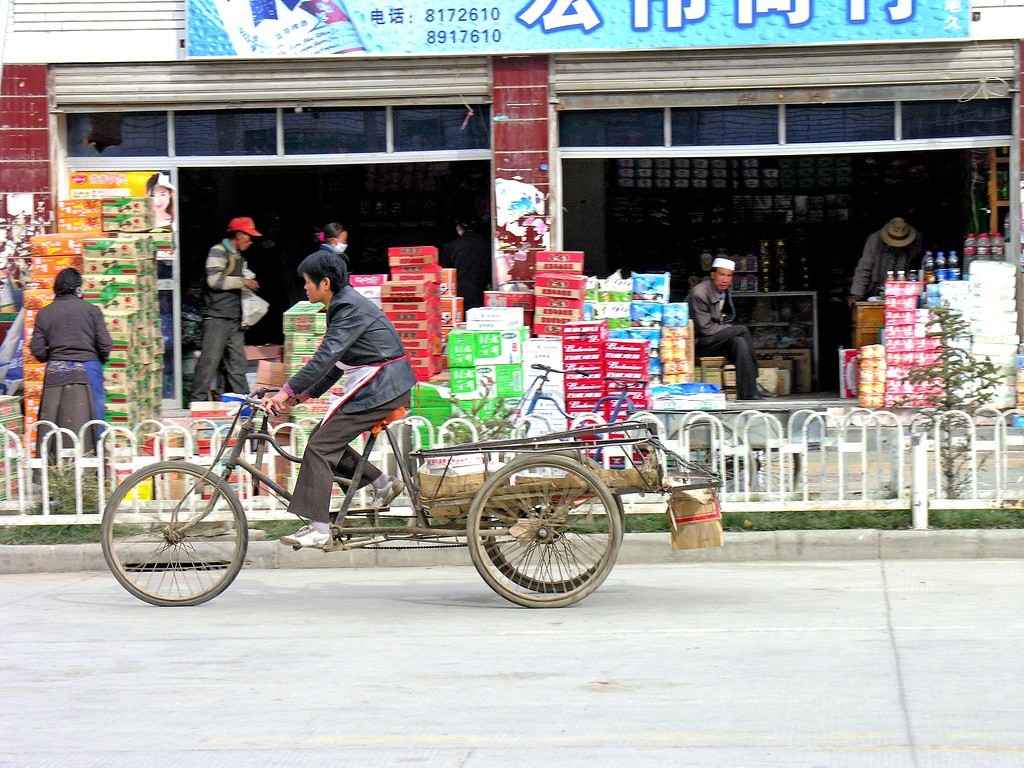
[880,218,916,247]
[147,173,173,192]
[228,217,263,236]
[712,258,736,270]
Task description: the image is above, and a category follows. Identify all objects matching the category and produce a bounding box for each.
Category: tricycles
[99,388,724,607]
[507,363,830,503]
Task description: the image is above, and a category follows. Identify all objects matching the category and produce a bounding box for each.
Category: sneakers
[279,524,333,549]
[368,476,406,508]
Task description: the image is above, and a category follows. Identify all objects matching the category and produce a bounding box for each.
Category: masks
[331,239,349,252]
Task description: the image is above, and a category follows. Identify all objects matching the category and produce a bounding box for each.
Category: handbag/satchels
[240,269,270,327]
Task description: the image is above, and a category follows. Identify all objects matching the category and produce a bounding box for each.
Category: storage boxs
[840,280,948,409]
[0,197,724,549]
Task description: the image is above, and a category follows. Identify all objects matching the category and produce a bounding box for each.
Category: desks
[850,301,885,348]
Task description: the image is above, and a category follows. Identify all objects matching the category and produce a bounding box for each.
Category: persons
[440,215,490,309]
[147,174,172,225]
[685,258,778,400]
[30,268,112,485]
[849,218,921,306]
[315,222,353,274]
[263,252,418,547]
[187,217,263,400]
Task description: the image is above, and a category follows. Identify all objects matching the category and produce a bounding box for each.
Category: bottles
[885,271,896,282]
[908,270,918,282]
[962,233,1004,278]
[896,271,907,281]
[1004,213,1010,240]
[924,254,935,286]
[921,251,931,271]
[935,251,947,282]
[948,251,961,280]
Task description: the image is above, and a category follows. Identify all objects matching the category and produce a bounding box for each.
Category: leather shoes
[755,383,777,397]
[747,394,765,400]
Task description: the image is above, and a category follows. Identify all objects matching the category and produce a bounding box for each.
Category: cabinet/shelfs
[729,288,820,387]
[990,145,1010,234]
